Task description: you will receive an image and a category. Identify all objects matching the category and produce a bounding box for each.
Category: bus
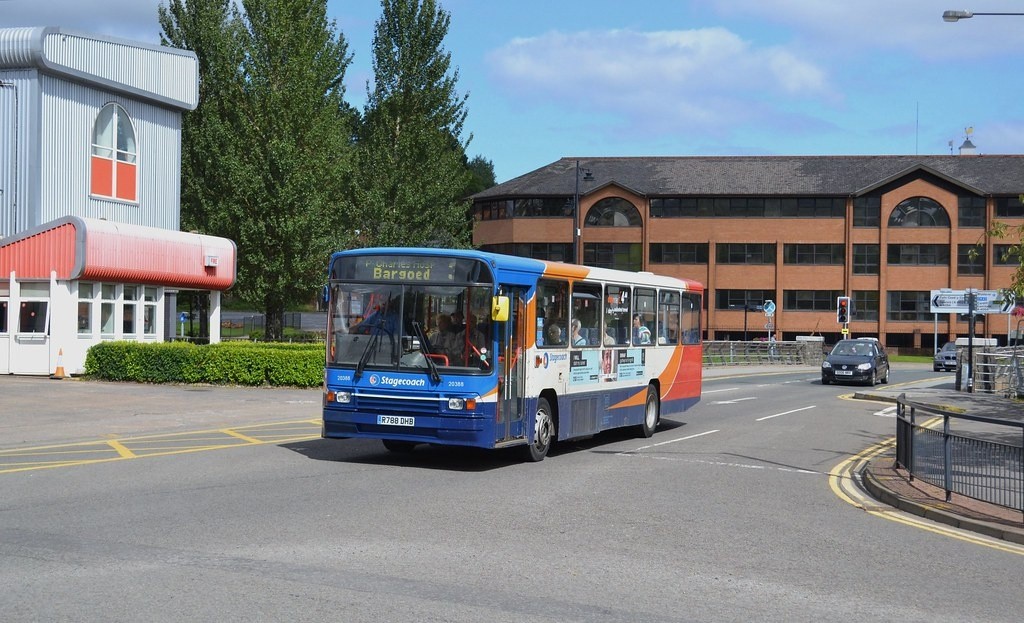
[321,248,705,464]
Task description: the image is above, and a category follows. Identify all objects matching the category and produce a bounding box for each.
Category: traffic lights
[837,297,849,322]
[850,299,857,316]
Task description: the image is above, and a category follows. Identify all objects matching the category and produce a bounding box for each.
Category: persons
[536,301,651,345]
[429,310,485,363]
[349,290,405,336]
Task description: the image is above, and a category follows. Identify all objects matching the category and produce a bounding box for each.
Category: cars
[821,339,890,386]
[933,340,964,372]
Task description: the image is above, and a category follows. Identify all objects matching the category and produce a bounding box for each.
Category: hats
[571,318,581,328]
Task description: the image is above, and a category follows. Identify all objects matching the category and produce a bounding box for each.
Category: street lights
[574,168,598,264]
[964,287,978,392]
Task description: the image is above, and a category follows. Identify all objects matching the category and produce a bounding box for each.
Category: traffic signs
[933,294,1011,311]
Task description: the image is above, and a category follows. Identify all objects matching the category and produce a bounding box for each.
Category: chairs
[588,328,615,345]
[562,328,590,345]
[646,320,663,343]
[611,319,627,344]
[451,324,466,333]
